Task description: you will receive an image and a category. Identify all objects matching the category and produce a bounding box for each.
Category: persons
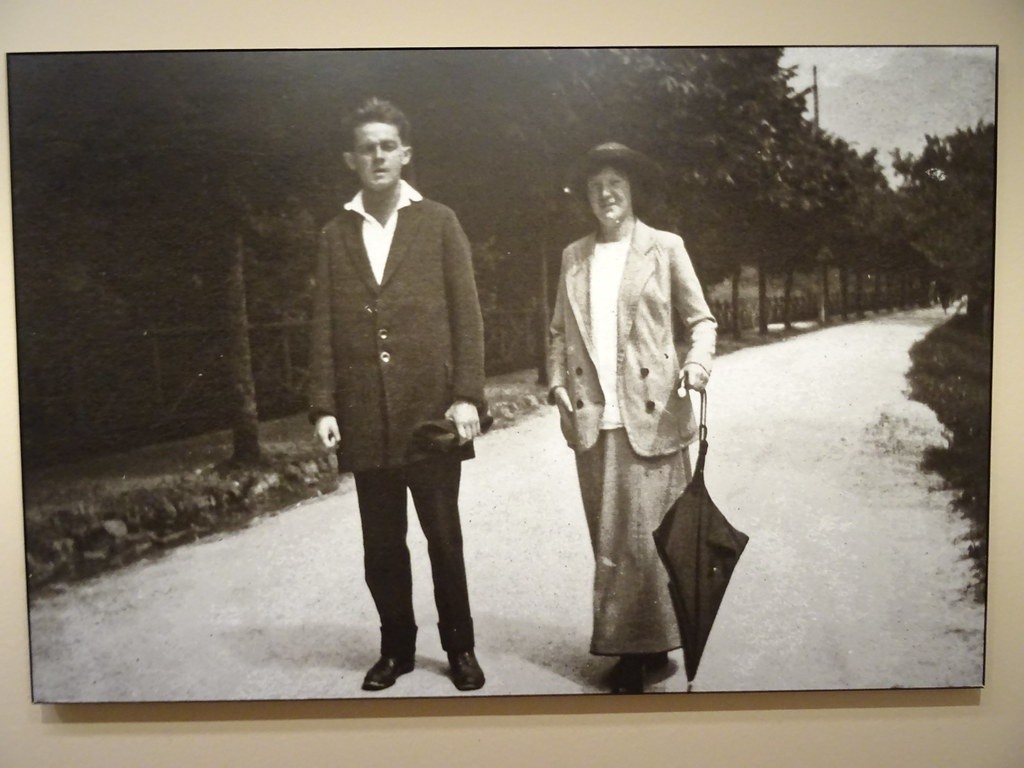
[539,140,720,694]
[305,95,487,692]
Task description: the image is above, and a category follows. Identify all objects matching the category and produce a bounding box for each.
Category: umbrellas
[651,369,749,691]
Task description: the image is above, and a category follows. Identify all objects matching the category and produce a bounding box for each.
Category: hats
[565,141,668,216]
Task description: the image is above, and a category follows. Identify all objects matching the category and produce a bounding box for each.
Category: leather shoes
[447,652,486,691]
[364,656,415,688]
